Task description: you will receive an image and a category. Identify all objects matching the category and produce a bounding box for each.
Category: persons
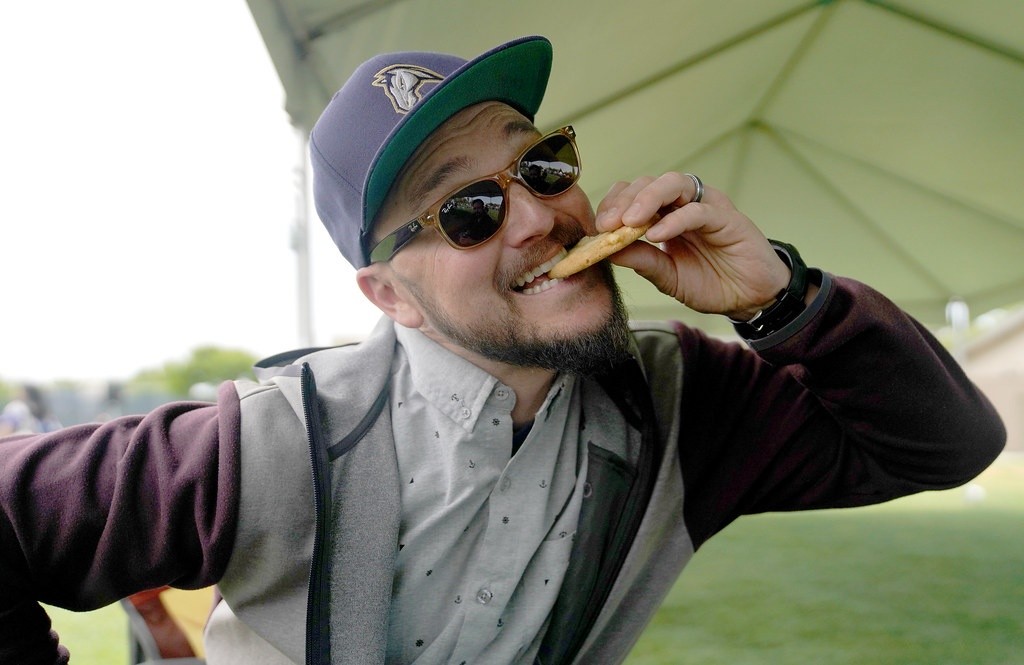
[0,34,1007,665]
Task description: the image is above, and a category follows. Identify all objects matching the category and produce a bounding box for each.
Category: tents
[245,0,1024,348]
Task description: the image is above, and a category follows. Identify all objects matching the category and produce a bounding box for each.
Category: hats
[309,35,553,271]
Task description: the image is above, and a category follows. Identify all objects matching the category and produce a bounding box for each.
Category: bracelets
[725,238,812,341]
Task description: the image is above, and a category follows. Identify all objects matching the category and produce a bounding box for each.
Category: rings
[683,173,704,203]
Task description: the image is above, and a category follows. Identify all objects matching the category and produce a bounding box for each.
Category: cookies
[548,213,659,279]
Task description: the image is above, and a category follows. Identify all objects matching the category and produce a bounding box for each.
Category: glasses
[366,125,583,265]
